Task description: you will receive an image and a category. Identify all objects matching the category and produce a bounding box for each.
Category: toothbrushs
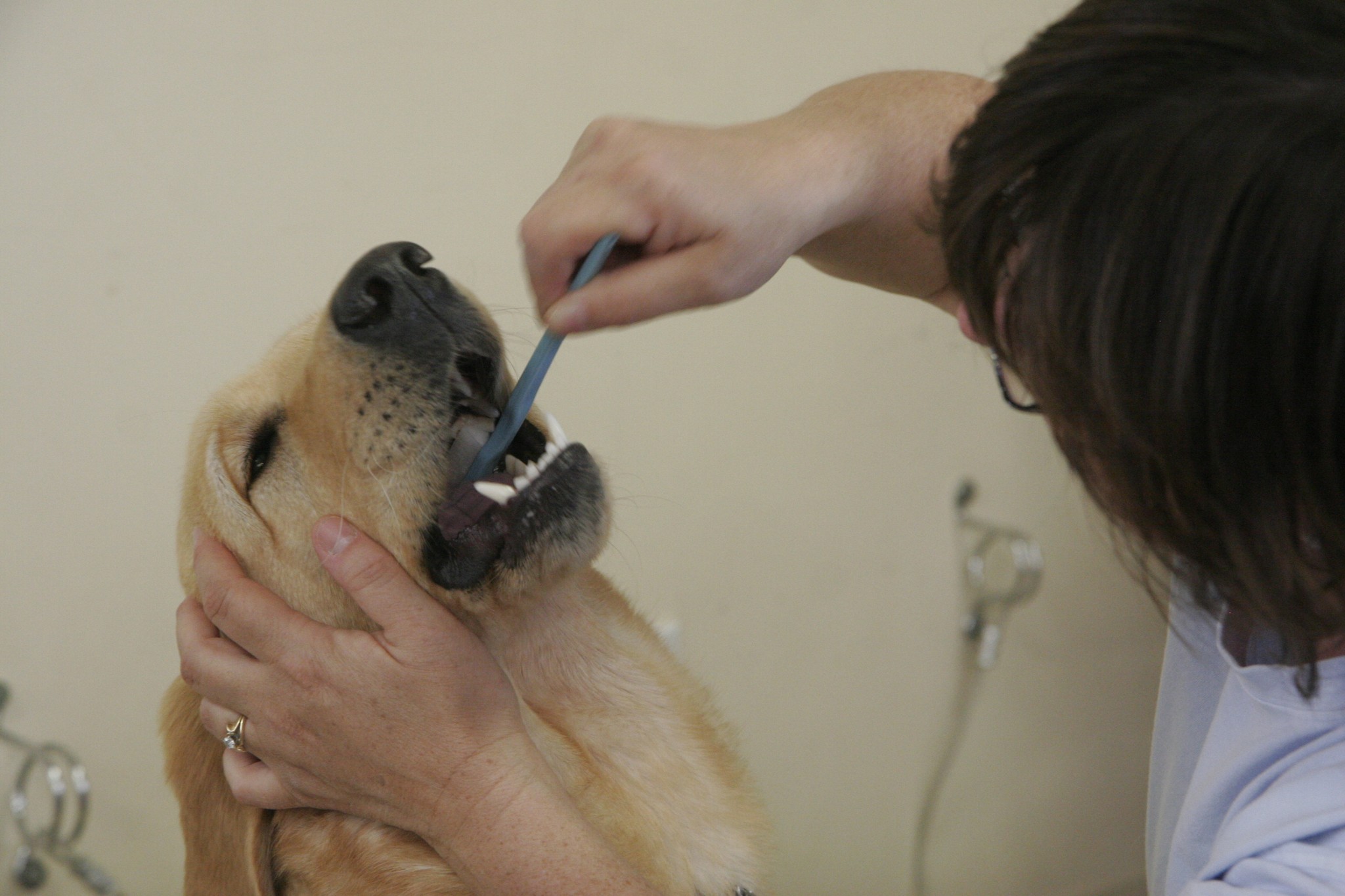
[445,231,621,487]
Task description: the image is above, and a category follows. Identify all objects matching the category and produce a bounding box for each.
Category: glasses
[991,357,1043,410]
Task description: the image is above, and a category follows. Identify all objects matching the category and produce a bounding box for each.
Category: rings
[221,714,249,755]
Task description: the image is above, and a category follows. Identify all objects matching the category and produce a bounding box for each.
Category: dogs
[151,235,785,896]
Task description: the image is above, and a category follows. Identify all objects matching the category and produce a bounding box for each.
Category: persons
[174,0,1345,896]
